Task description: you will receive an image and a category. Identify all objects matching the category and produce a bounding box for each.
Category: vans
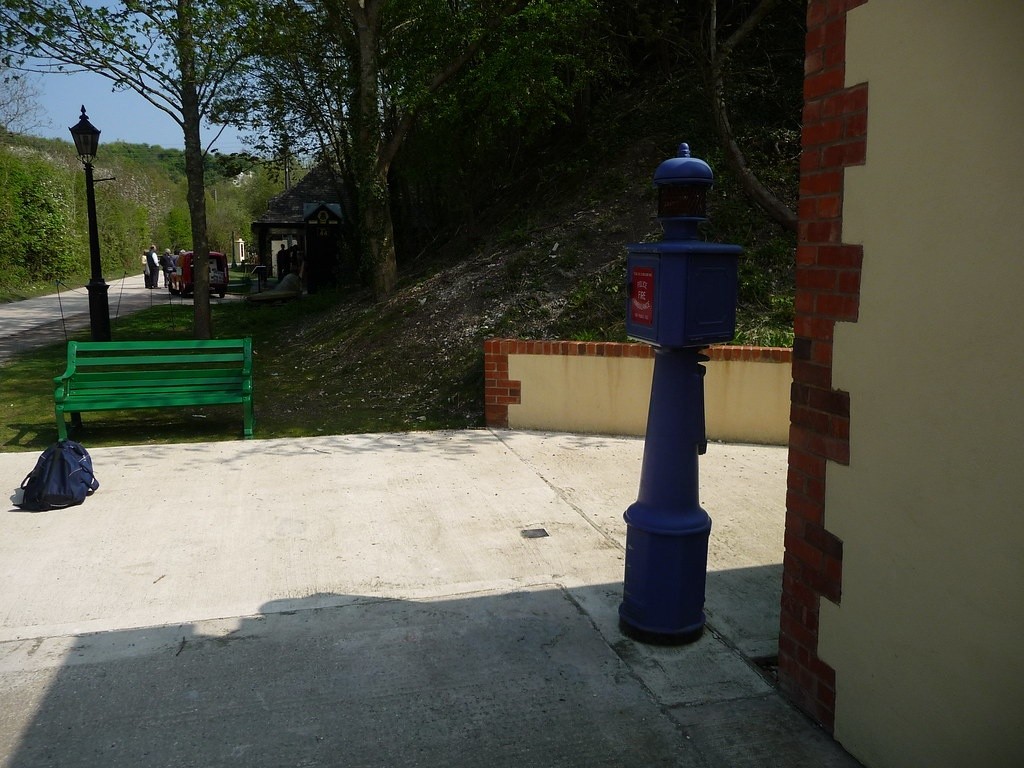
[168,249,230,298]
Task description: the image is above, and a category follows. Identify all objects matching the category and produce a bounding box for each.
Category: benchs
[53,337,254,442]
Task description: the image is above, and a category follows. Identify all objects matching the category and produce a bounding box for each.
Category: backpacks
[13,439,100,511]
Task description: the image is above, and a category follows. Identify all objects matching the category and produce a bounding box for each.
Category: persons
[141,245,184,289]
[277,243,298,282]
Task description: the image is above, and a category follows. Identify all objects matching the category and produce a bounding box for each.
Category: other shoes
[145,286,161,289]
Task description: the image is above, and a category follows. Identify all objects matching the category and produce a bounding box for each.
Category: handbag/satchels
[145,263,150,276]
[168,267,173,271]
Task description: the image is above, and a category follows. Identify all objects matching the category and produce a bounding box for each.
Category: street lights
[67,105,113,341]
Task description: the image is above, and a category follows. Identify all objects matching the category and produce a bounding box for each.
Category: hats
[165,248,170,252]
[181,250,185,252]
